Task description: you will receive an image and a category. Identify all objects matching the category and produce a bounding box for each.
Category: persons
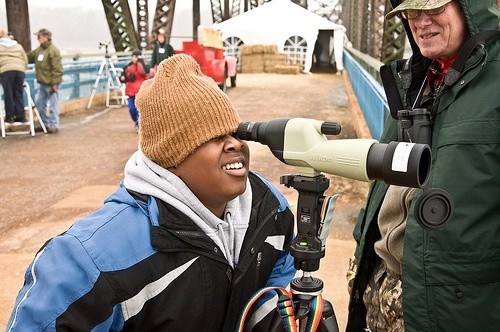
[147,35,178,79]
[118,51,149,135]
[343,1,499,332]
[6,52,299,332]
[0,30,28,124]
[26,29,62,134]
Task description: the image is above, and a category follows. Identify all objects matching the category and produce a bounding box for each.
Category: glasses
[401,6,445,20]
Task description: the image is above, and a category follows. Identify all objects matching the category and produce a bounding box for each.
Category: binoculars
[398,108,431,148]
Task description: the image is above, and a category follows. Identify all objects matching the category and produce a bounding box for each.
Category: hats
[384,0,452,20]
[134,53,241,170]
[33,28,52,37]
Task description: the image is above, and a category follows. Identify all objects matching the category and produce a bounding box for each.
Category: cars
[174,41,239,93]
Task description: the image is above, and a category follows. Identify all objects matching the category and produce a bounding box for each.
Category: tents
[197,0,346,76]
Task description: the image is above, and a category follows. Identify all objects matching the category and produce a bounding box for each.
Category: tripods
[269,174,340,332]
[86,44,129,109]
